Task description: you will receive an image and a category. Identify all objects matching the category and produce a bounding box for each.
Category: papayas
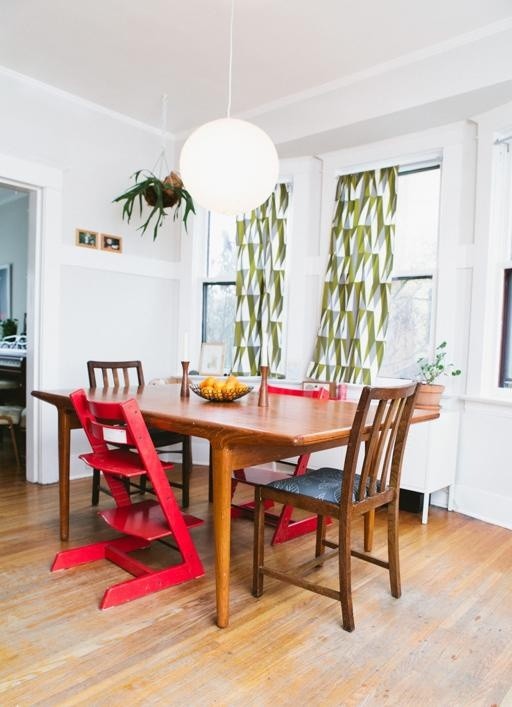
[199,376,248,401]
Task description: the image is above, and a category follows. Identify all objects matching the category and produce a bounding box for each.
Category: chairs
[231,383,332,546]
[88,361,191,508]
[252,382,419,632]
[54,388,207,611]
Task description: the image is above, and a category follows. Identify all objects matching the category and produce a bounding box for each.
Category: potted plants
[412,340,462,411]
[111,167,196,241]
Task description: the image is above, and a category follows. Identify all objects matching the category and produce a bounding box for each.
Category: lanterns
[179,118,280,213]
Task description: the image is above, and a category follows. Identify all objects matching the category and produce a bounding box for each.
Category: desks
[30,383,440,627]
